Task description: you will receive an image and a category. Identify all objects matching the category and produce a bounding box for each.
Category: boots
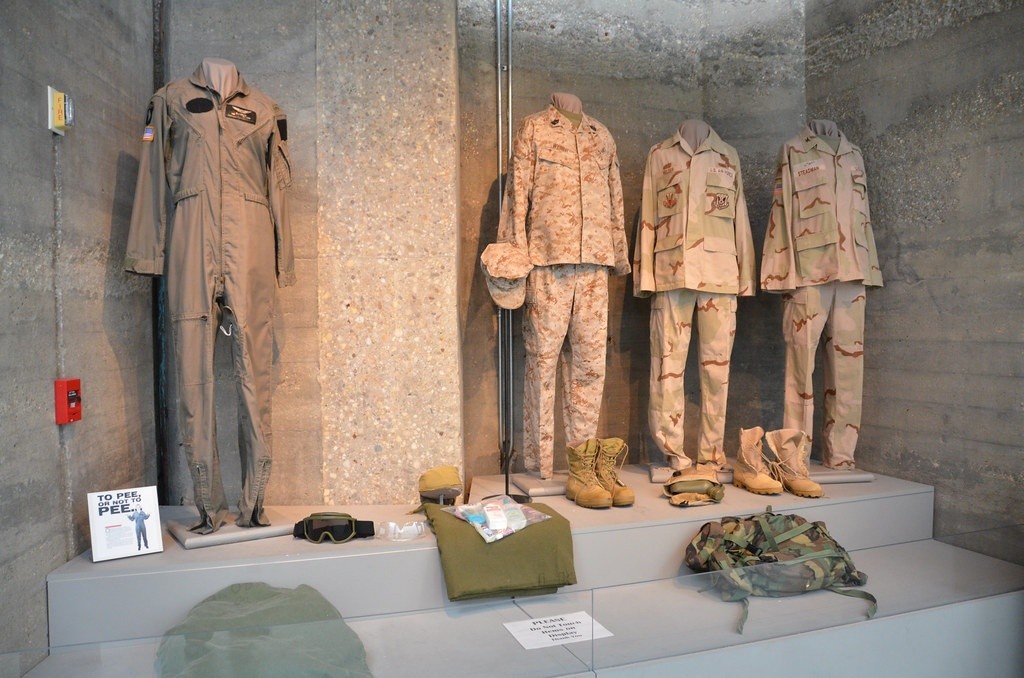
[595,436,635,507]
[565,438,613,509]
[733,425,783,495]
[765,428,824,498]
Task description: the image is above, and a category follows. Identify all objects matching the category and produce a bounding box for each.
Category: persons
[124,58,299,535]
[633,119,757,471]
[127,504,151,550]
[760,120,884,471]
[481,91,632,479]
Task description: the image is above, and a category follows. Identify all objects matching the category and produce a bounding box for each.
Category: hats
[481,242,535,309]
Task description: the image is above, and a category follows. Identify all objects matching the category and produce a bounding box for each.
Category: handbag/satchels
[684,506,878,634]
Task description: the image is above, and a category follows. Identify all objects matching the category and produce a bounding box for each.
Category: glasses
[303,511,355,544]
